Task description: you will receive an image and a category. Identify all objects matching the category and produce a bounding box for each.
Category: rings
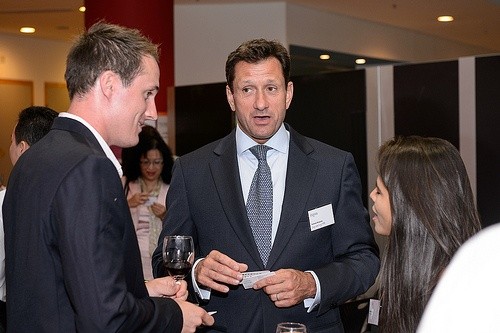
[275,294,280,301]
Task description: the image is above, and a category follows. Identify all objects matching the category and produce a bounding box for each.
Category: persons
[121,139,174,288]
[152,38,380,333]
[118,122,164,181]
[2,20,215,333]
[370,136,483,333]
[0,105,61,302]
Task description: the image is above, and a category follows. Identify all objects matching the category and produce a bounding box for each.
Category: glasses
[141,158,164,169]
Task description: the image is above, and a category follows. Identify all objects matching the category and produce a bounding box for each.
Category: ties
[245,145,275,270]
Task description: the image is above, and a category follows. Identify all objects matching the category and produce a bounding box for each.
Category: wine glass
[162,236,194,298]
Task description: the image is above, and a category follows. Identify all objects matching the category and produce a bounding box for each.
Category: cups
[276,323,306,333]
